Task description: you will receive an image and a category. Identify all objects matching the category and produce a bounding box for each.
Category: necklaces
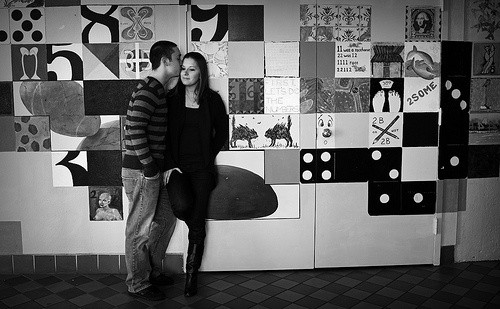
[185,87,201,107]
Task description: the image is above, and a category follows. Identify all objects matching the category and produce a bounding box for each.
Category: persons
[120,38,183,302]
[164,51,229,299]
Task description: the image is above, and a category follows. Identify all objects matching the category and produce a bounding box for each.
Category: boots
[184,241,204,297]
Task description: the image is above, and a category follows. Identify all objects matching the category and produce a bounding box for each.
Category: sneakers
[149,272,176,286]
[128,282,166,301]
